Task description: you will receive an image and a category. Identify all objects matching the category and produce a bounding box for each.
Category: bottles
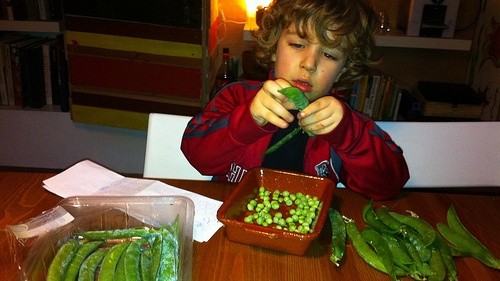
[215,47,232,92]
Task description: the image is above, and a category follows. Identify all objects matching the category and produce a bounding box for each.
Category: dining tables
[0,165,500,281]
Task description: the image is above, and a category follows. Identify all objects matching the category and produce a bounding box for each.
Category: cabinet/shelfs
[0,0,486,176]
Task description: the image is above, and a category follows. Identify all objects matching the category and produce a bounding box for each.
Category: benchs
[143,111,500,188]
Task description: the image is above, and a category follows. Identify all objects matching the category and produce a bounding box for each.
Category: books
[330,69,485,122]
[0,0,69,112]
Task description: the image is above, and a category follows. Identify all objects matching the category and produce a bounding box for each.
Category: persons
[180,0,410,201]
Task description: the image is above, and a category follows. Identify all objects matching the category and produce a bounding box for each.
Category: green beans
[244,186,320,234]
[47,212,179,281]
[260,87,314,156]
[326,198,500,281]
